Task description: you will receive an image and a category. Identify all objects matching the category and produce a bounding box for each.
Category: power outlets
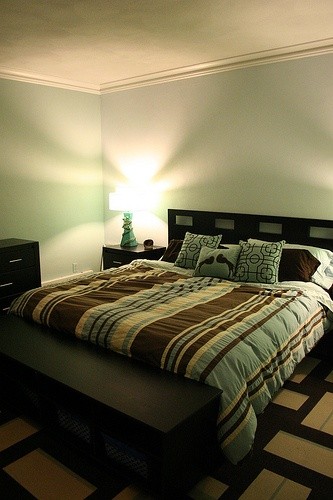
[73,263,77,272]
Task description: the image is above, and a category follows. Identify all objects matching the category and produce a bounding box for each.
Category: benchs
[0,310,223,500]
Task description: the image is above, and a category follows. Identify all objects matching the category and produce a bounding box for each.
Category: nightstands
[103,243,166,270]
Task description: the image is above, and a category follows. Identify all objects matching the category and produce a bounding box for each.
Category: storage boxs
[21,381,42,410]
[103,433,155,481]
[55,405,91,441]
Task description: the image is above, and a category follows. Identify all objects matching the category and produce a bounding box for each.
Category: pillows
[233,240,287,284]
[248,238,333,291]
[278,248,321,283]
[174,231,223,270]
[192,245,244,280]
[161,238,230,263]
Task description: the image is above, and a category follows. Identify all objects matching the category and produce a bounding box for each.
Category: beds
[5,208,333,467]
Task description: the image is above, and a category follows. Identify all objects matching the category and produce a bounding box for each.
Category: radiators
[41,270,93,288]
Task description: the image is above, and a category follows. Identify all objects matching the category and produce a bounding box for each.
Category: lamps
[108,185,148,248]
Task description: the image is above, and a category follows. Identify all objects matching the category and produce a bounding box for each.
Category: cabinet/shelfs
[0,237,42,309]
